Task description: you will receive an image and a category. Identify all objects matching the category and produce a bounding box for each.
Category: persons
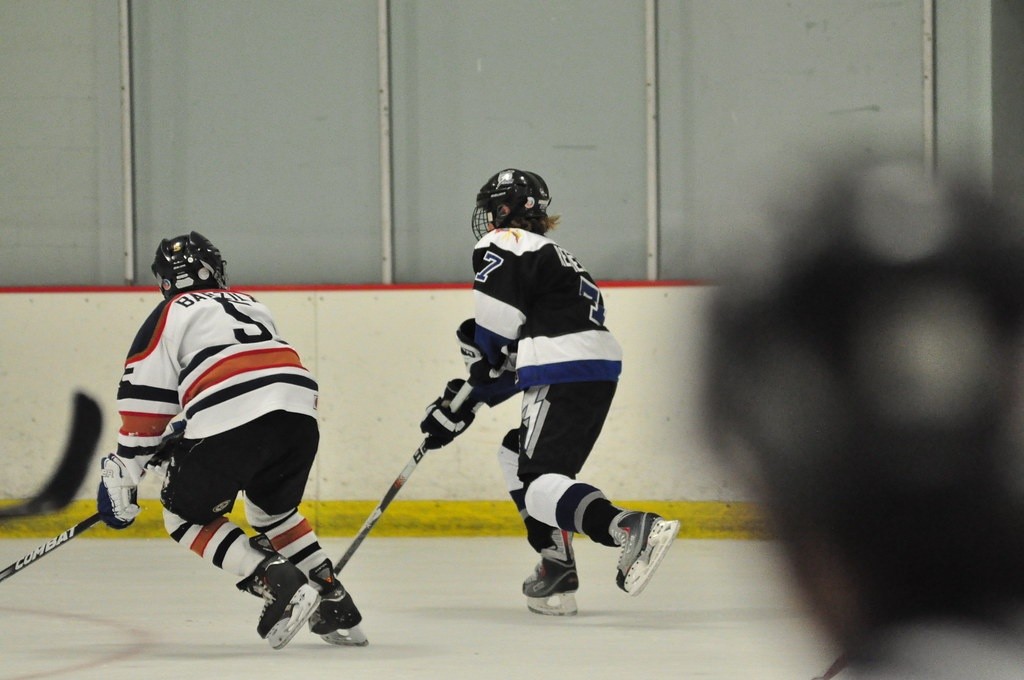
[419,169,680,617]
[97,230,369,650]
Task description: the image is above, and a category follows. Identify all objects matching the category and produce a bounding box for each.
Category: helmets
[471,167,552,242]
[151,230,225,298]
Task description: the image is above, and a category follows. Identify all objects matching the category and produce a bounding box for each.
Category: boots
[523,527,579,597]
[302,559,362,636]
[234,533,308,640]
[608,503,665,593]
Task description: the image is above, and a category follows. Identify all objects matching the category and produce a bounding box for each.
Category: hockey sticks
[1,512,103,584]
[1,386,104,521]
[331,374,475,577]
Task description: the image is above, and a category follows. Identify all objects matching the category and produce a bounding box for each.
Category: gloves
[455,318,507,385]
[142,420,187,476]
[98,455,141,530]
[419,379,485,450]
